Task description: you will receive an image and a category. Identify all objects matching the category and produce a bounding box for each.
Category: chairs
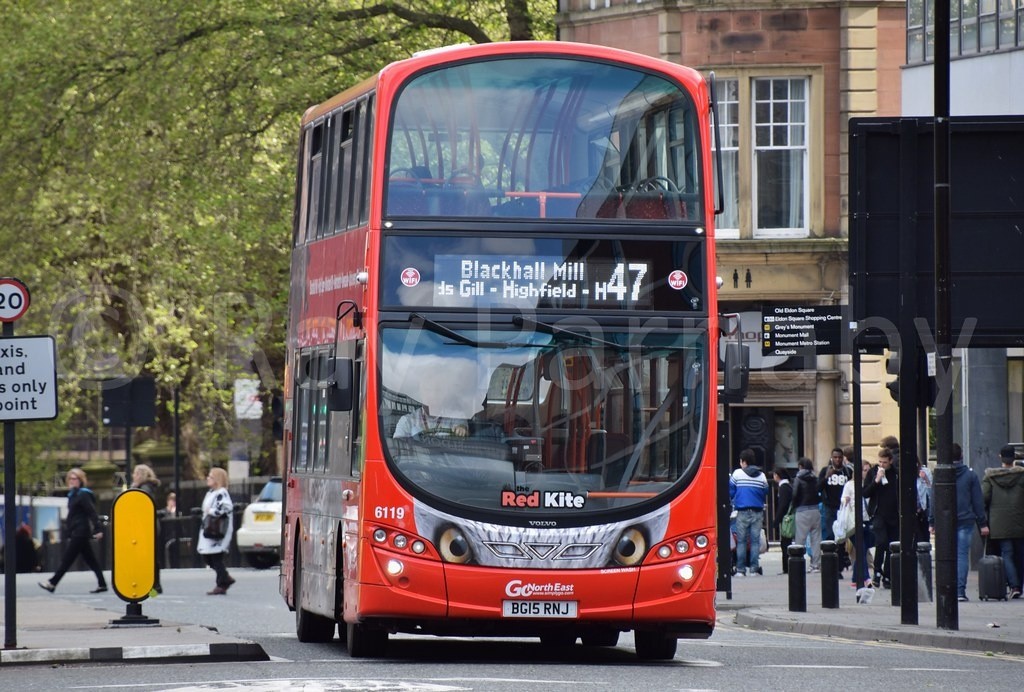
[388,166,690,219]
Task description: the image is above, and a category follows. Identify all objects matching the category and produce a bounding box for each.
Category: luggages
[977,533,1008,601]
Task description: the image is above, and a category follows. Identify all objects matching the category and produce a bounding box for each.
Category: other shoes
[90,586,107,592]
[37,580,56,592]
[734,573,743,577]
[812,565,820,572]
[750,572,759,576]
[874,572,881,588]
[883,577,891,588]
[865,578,873,587]
[206,576,235,595]
[850,583,857,588]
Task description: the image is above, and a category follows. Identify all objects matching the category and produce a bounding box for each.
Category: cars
[235,476,284,567]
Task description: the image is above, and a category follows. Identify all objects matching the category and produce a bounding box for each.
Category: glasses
[68,475,78,480]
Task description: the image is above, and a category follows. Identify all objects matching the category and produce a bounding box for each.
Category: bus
[275,35,752,669]
[0,493,70,574]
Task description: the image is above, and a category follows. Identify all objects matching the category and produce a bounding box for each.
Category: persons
[197,467,236,595]
[819,447,853,582]
[789,459,822,571]
[928,441,989,601]
[843,448,855,471]
[38,467,108,594]
[979,445,1024,600]
[727,448,770,577]
[167,493,177,515]
[863,449,899,589]
[879,436,899,471]
[832,460,872,587]
[129,464,164,596]
[770,467,794,574]
[915,454,933,541]
[393,374,469,440]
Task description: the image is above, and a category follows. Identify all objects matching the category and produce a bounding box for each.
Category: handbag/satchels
[203,515,229,538]
[782,515,796,539]
[831,496,857,544]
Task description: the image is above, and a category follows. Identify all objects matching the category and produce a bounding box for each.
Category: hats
[1000,446,1015,457]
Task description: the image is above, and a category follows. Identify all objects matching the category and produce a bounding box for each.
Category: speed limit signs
[0,279,29,323]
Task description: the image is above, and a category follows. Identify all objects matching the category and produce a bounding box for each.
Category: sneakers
[958,596,968,601]
[1009,588,1020,599]
